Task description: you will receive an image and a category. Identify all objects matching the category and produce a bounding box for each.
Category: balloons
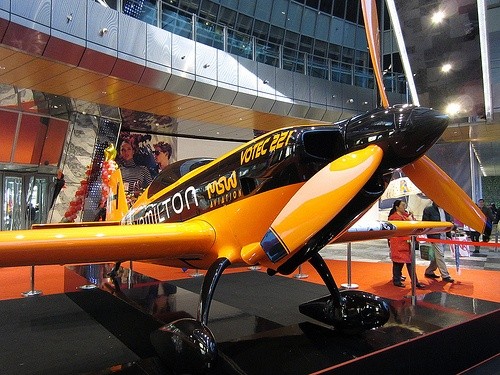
[61,169,92,223]
[100,143,118,208]
[57,169,64,179]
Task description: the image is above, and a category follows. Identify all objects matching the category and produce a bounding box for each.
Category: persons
[478,198,492,242]
[451,220,457,237]
[489,203,500,224]
[422,200,454,282]
[466,231,480,253]
[116,132,172,207]
[387,199,426,287]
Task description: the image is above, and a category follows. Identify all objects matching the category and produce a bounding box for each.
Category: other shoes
[473,250,480,253]
[400,277,405,281]
[401,275,406,279]
[416,282,425,288]
[425,273,440,278]
[443,276,454,282]
[488,237,491,242]
[395,282,405,287]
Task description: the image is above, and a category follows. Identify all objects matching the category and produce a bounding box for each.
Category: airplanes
[0,0,500,375]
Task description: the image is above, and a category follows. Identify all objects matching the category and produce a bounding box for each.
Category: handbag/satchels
[420,244,435,261]
